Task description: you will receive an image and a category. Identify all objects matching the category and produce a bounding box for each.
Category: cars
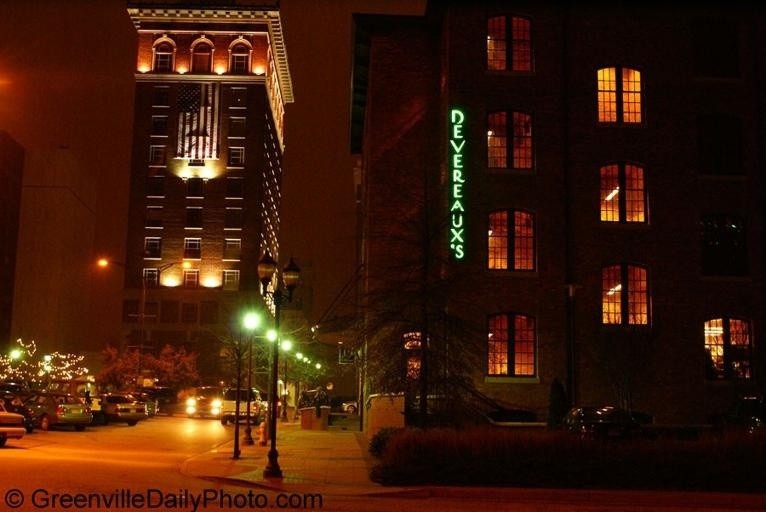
[341,399,358,415]
[186,386,223,419]
[1,379,177,447]
[220,387,261,425]
[548,404,648,448]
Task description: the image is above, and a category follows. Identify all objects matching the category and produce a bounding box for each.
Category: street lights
[242,311,261,445]
[280,339,293,423]
[257,249,302,478]
[265,329,277,440]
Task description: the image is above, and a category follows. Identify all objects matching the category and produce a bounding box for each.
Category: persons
[85,389,94,408]
[296,384,330,418]
